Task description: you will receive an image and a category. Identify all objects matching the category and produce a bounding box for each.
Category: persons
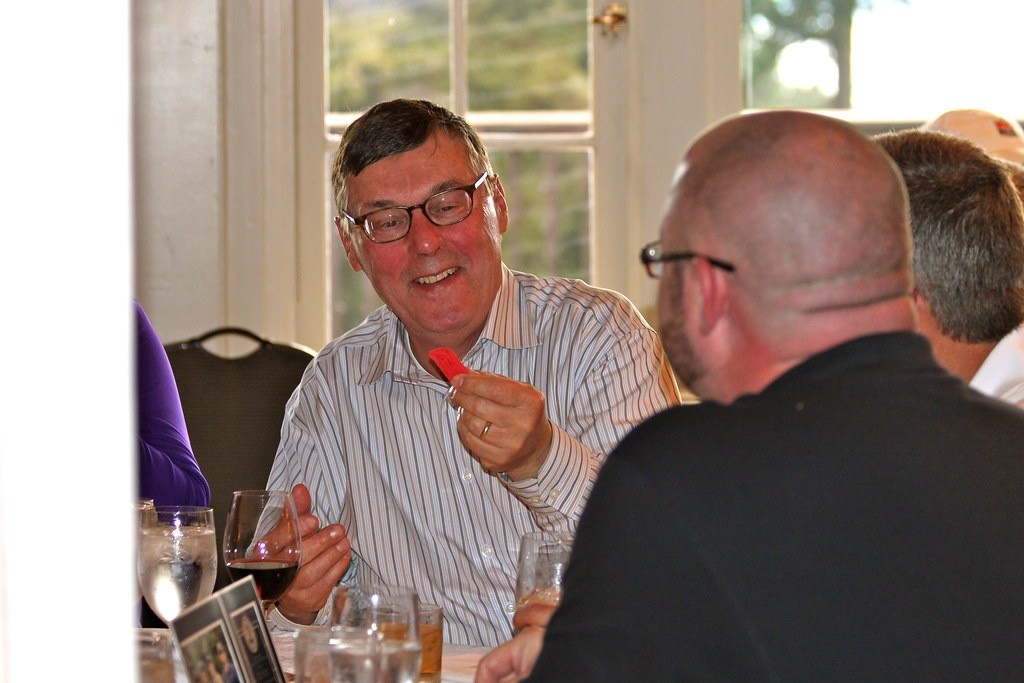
[918,108,1024,202]
[135,297,210,525]
[245,99,685,650]
[478,421,493,440]
[476,99,1024,682]
[512,130,1024,630]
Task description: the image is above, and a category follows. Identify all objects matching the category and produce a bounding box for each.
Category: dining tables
[138,627,499,683]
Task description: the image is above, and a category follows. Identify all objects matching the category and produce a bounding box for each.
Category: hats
[921,109,1024,174]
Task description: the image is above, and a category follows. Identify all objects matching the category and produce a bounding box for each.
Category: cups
[327,584,422,683]
[514,531,576,607]
[372,602,444,683]
[136,496,158,602]
[291,624,329,683]
[136,504,219,631]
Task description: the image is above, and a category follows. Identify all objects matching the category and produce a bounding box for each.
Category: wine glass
[222,490,302,620]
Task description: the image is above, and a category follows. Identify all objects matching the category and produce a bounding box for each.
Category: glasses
[339,172,492,243]
[640,241,735,279]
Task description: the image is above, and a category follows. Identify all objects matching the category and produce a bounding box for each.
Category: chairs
[162,326,319,597]
